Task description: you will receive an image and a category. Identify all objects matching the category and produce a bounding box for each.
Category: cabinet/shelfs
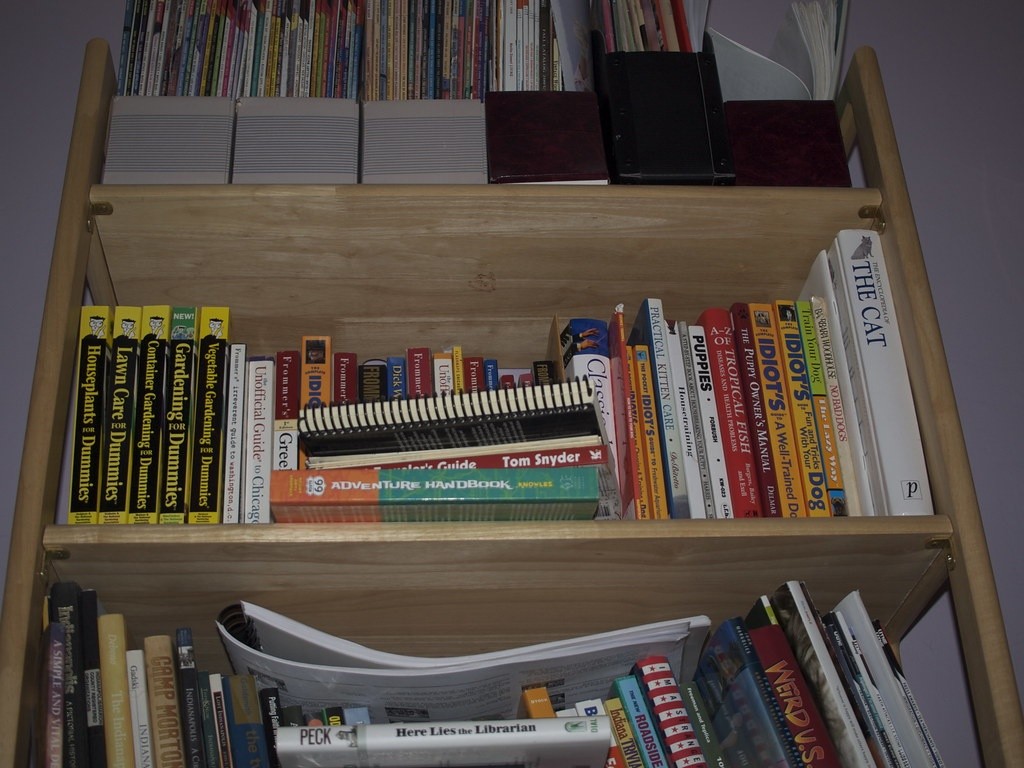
[0,37,1024,768]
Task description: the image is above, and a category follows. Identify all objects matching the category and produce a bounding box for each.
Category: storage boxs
[725,99,853,187]
[590,28,737,186]
[484,90,610,184]
[362,100,489,185]
[103,95,237,185]
[232,97,360,185]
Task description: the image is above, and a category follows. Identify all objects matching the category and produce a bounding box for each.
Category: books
[53,298,862,524]
[115,0,851,102]
[797,228,934,515]
[29,582,946,768]
